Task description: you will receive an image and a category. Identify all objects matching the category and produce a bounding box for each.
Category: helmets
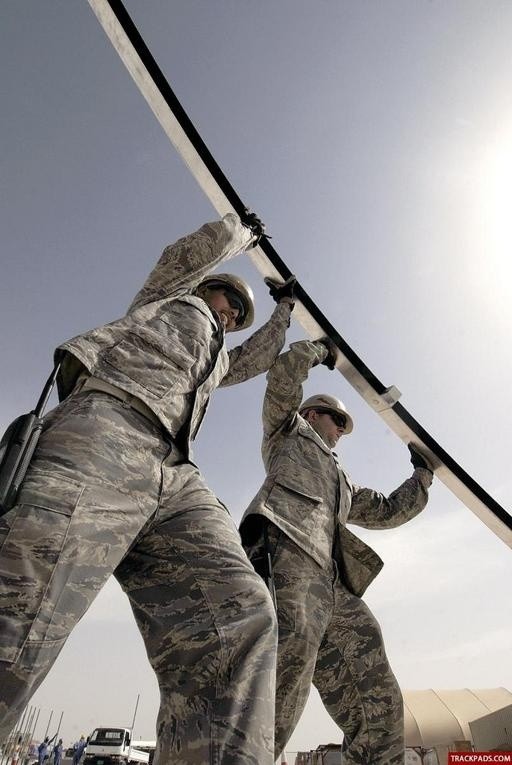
[204,271,255,334]
[302,395,354,435]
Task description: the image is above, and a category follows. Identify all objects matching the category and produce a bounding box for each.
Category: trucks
[84,727,150,765]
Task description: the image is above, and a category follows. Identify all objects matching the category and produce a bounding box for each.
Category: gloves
[407,443,436,476]
[319,342,336,372]
[265,275,296,309]
[244,210,267,251]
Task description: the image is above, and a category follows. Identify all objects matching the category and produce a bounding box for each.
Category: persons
[0,206,298,765]
[72,733,90,765]
[26,741,40,765]
[12,736,22,765]
[53,739,67,765]
[38,735,50,765]
[237,331,439,765]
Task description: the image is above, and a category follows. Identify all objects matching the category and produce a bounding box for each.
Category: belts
[70,379,168,423]
